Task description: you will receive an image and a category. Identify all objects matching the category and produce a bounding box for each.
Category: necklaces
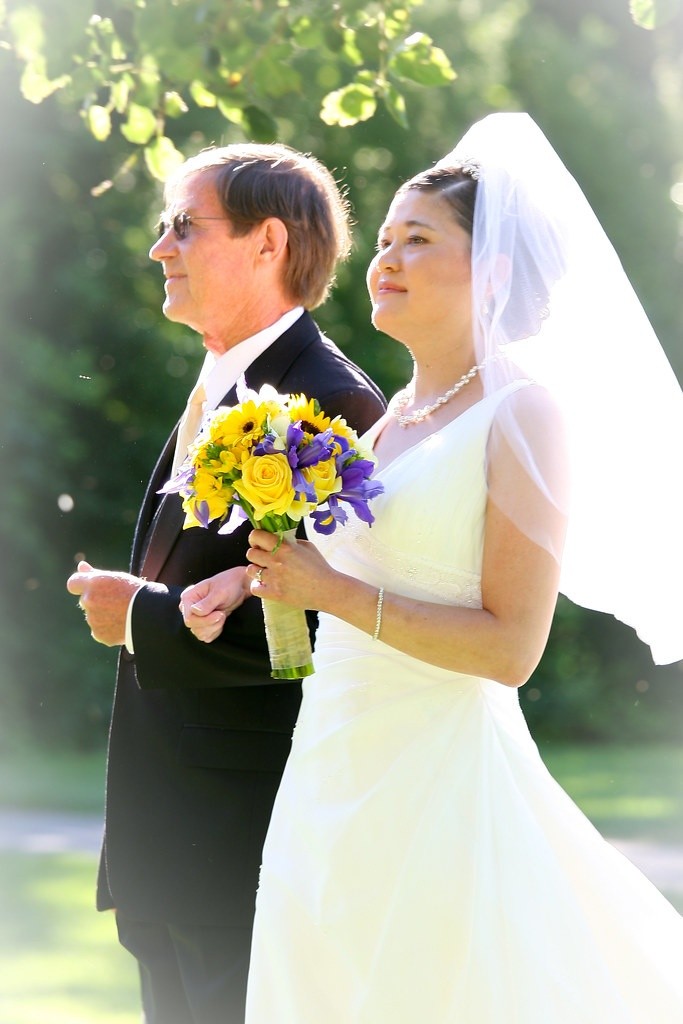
[391,349,508,429]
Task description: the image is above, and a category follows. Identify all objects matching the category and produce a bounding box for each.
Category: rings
[255,567,264,585]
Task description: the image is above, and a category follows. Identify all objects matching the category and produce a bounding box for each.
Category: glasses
[157,213,230,241]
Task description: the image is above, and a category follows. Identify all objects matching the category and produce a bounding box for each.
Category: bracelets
[372,588,385,641]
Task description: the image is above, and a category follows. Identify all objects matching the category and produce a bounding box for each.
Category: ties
[170,384,206,480]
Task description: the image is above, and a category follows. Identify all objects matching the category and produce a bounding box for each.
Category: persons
[65,141,386,1023]
[177,161,683,1023]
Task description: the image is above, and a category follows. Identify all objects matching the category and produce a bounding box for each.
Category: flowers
[154,382,387,679]
